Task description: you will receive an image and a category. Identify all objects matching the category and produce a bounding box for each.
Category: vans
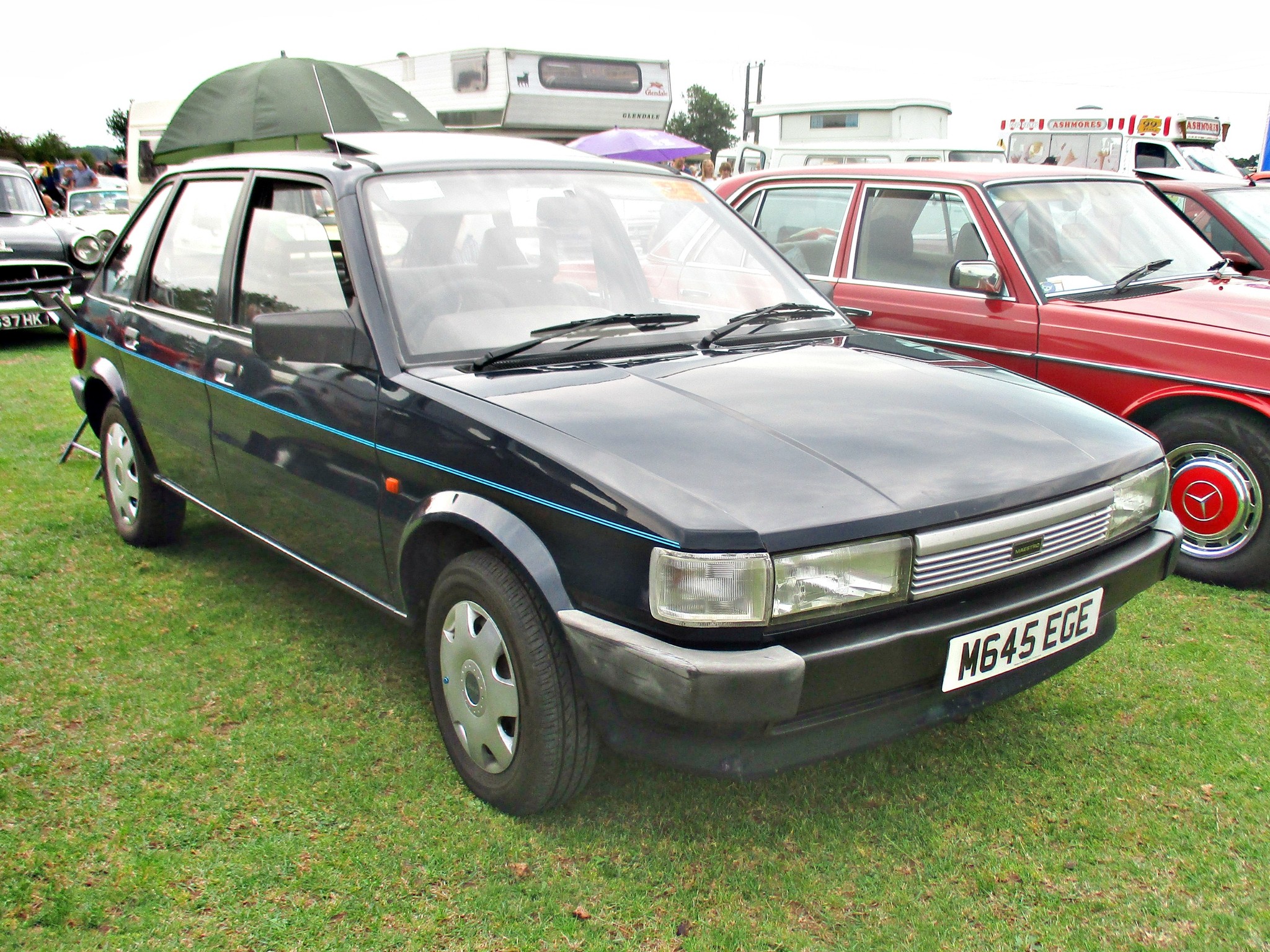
[734,142,1006,177]
[1001,117,1251,186]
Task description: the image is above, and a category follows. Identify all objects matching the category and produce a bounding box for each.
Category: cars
[553,164,1270,589]
[68,130,1184,818]
[912,164,1270,280]
[0,161,108,336]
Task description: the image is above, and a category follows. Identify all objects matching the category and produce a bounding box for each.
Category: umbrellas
[154,51,444,165]
[565,125,711,161]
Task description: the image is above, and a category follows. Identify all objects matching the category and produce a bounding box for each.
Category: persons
[245,301,275,324]
[105,154,154,181]
[34,156,98,209]
[755,162,762,170]
[666,156,732,192]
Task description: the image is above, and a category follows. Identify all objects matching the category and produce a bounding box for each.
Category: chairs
[945,223,988,292]
[861,216,936,289]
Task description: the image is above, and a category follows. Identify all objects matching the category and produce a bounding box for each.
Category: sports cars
[54,188,132,252]
